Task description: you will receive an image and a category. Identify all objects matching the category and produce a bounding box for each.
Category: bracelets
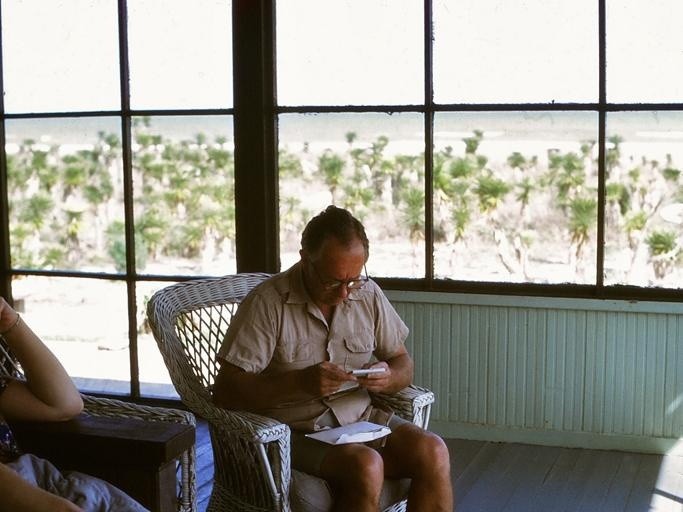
[2,314,20,339]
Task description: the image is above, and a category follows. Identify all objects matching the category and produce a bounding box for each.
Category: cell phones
[347,368,385,378]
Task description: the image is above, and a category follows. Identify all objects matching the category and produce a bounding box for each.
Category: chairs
[146,271,436,511]
[0,334,198,512]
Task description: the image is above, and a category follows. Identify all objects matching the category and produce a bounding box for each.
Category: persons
[212,205,454,512]
[0,296,153,512]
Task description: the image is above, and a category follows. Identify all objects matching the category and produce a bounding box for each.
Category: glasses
[309,259,369,289]
